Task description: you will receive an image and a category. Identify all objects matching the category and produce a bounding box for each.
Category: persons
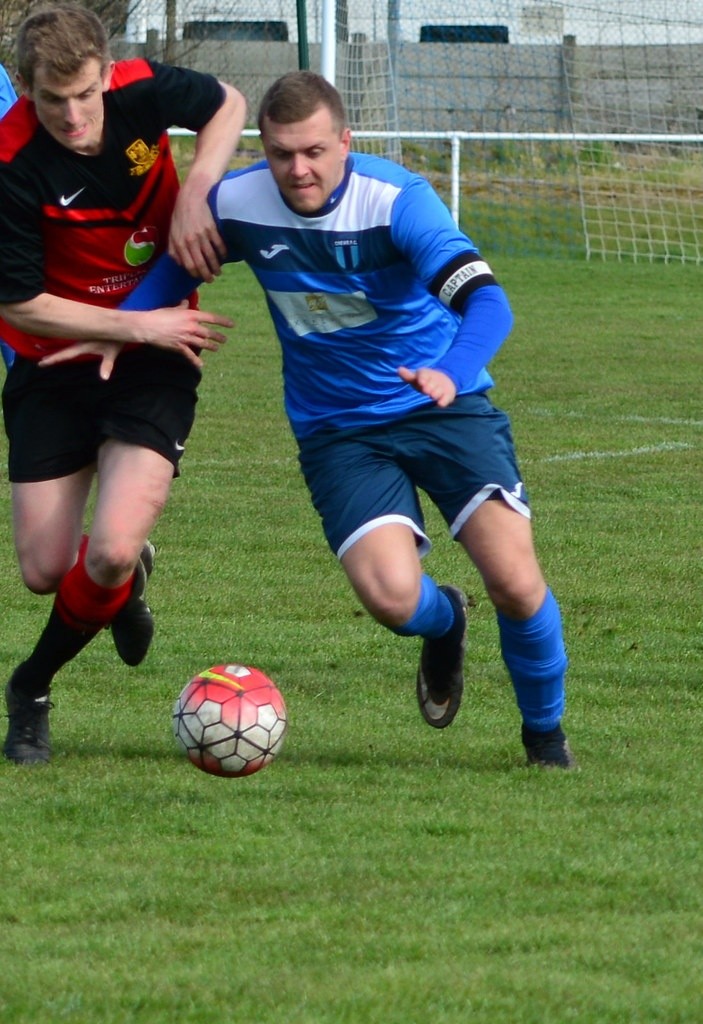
[0,3,246,761]
[0,61,21,369]
[38,67,569,767]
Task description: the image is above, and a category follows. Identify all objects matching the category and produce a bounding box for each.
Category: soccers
[173,663,289,779]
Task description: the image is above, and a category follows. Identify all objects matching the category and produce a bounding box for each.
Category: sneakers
[103,538,155,666]
[416,585,466,728]
[521,724,574,776]
[5,662,54,769]
